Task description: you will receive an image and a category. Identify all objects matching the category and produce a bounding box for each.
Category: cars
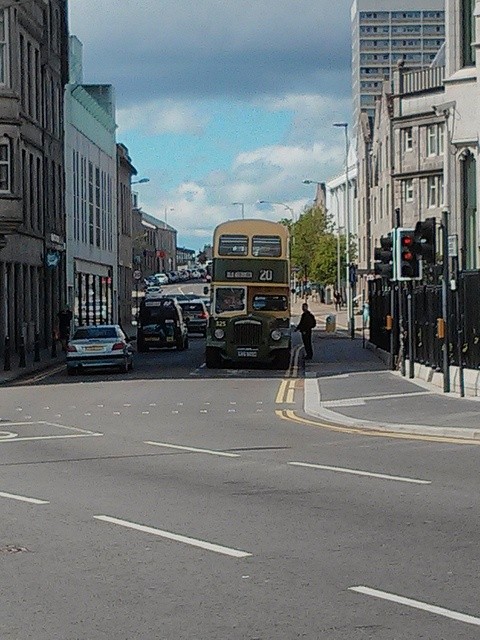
[155,272,168,285]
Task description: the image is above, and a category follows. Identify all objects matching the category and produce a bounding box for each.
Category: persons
[56,305,73,353]
[293,303,316,360]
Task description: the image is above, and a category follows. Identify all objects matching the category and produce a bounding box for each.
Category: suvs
[134,296,191,353]
[65,325,135,376]
[178,298,211,340]
[144,286,163,299]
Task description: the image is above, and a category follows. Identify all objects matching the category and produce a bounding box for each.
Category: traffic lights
[412,217,436,265]
[373,247,383,275]
[396,227,423,281]
[380,227,396,281]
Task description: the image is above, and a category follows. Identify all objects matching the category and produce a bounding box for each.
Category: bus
[205,218,297,371]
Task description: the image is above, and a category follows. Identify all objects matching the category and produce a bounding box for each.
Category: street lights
[131,177,150,186]
[256,199,297,303]
[333,122,353,335]
[301,180,341,311]
[232,202,244,220]
[164,206,176,229]
